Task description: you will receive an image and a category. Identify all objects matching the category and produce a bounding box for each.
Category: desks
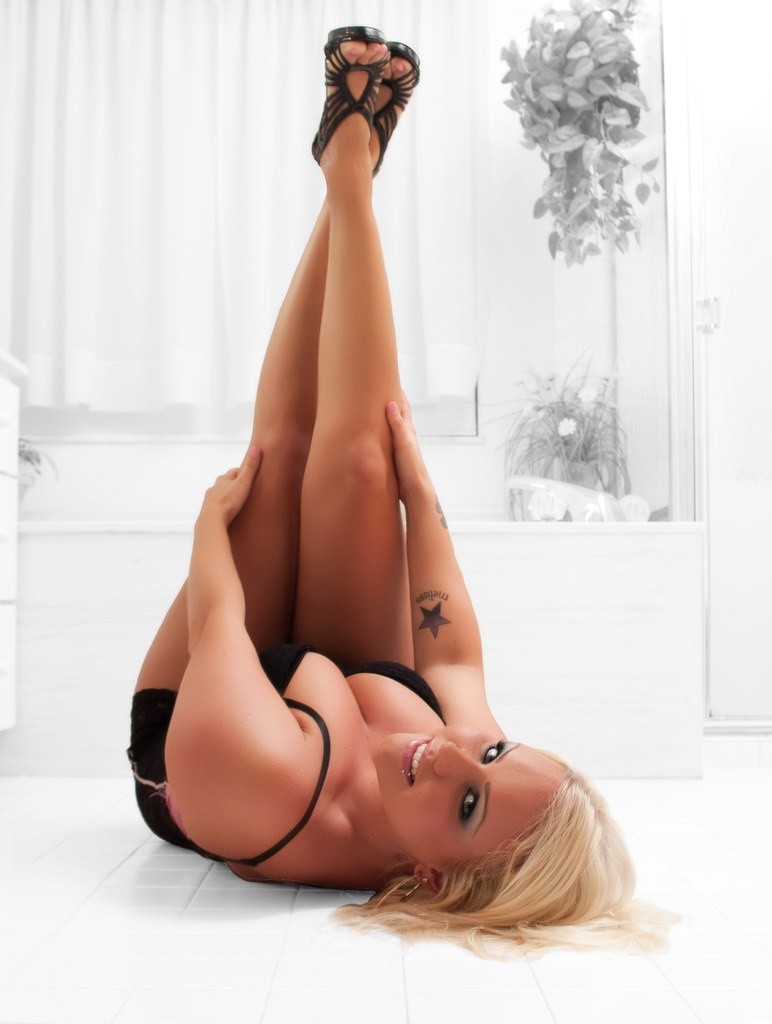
[442,518,716,789]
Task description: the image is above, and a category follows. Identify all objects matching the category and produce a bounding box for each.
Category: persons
[127,27,658,958]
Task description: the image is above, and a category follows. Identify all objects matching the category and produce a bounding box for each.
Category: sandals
[311,26,390,166]
[372,42,420,177]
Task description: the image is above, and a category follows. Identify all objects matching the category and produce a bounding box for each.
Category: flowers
[502,364,633,495]
